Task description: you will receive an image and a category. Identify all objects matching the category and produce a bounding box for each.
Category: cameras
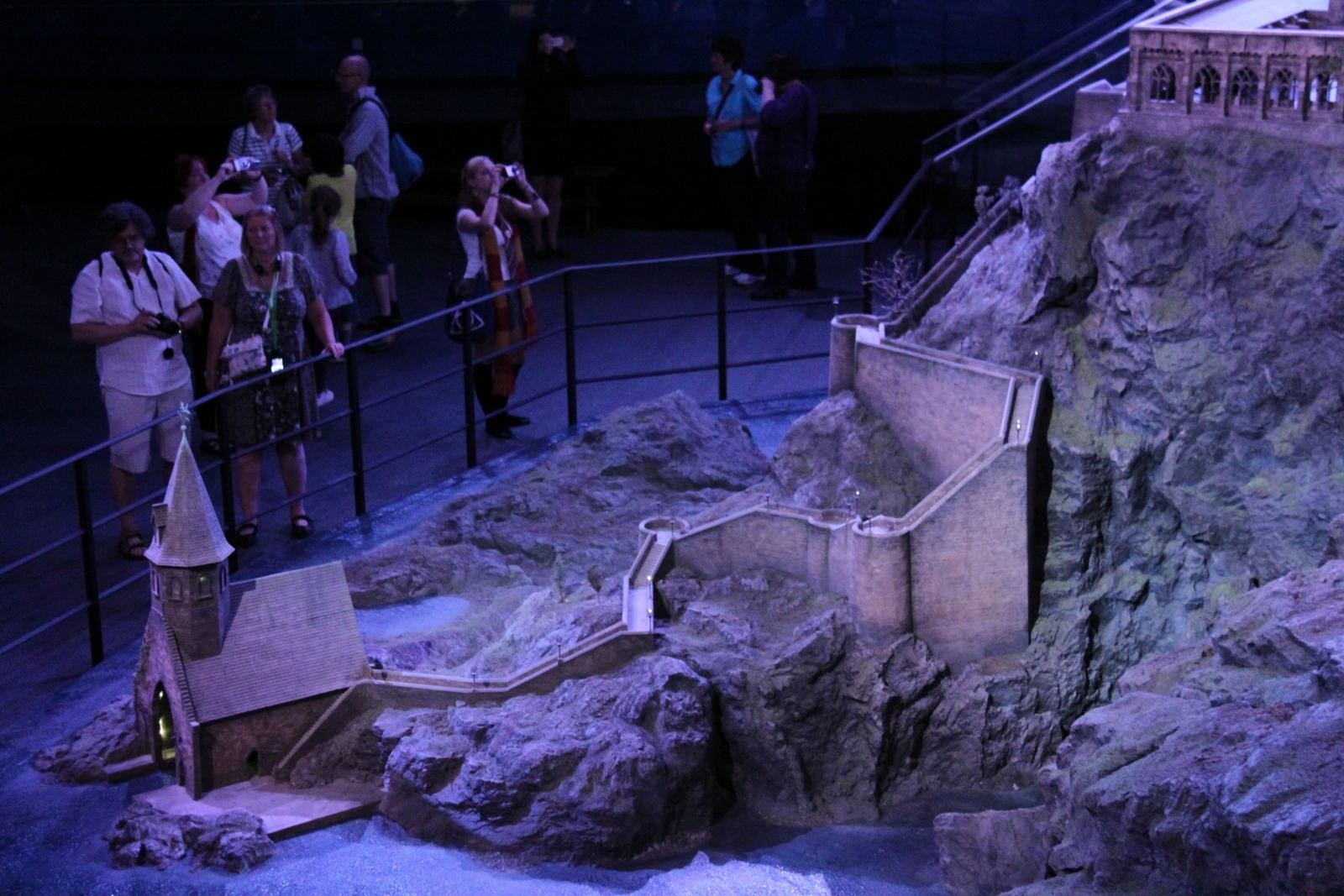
[150,313,181,340]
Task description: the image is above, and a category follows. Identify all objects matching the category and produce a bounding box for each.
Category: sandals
[120,530,150,561]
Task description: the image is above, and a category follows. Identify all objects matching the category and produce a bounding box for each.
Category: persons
[227,83,312,231]
[302,134,359,257]
[333,53,409,336]
[69,203,205,561]
[513,31,581,267]
[750,48,825,301]
[161,154,274,458]
[454,155,549,443]
[697,33,768,290]
[199,204,353,545]
[284,181,357,408]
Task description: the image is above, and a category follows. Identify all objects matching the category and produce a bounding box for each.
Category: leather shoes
[237,521,258,547]
[290,516,315,537]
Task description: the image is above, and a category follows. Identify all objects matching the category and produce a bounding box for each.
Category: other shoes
[724,263,817,301]
[486,414,531,440]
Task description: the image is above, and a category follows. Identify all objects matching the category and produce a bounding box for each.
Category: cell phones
[234,156,251,173]
[499,165,521,179]
[267,354,284,375]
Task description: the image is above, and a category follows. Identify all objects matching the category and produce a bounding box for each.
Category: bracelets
[527,191,540,202]
[488,192,499,199]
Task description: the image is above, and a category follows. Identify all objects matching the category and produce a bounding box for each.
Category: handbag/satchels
[276,179,305,232]
[220,335,266,382]
[387,132,424,192]
[446,267,497,344]
